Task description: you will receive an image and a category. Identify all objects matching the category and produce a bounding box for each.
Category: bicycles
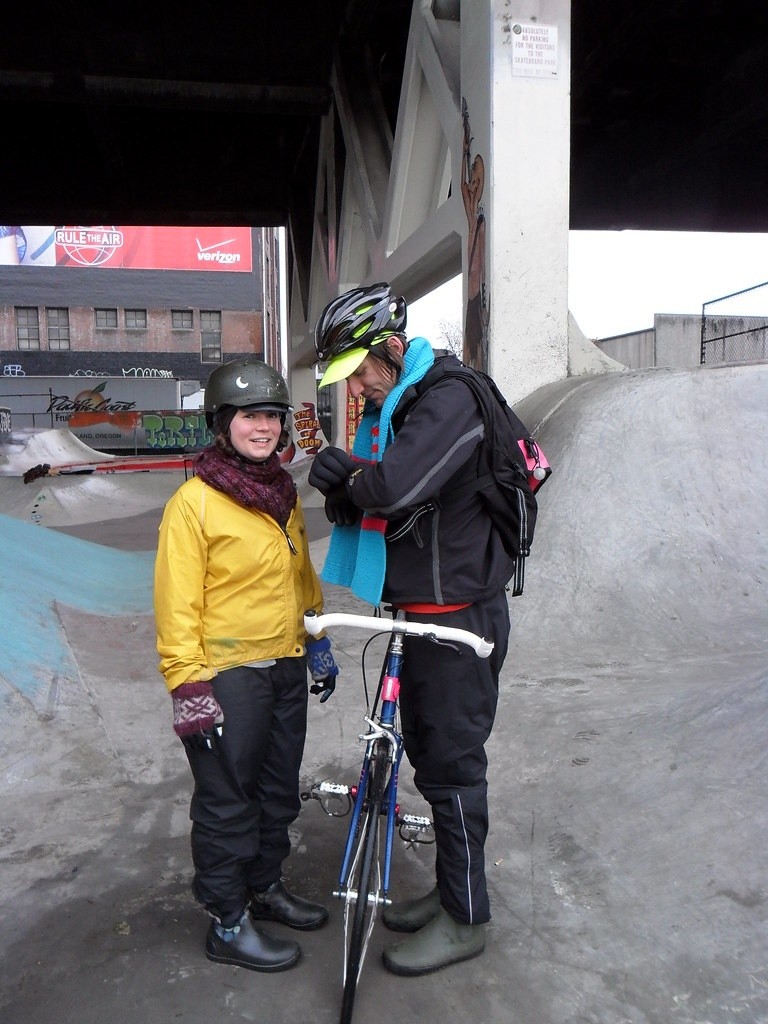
[298,605,496,1024]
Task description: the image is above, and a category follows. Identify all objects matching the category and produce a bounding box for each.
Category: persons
[154,358,340,972]
[308,282,515,976]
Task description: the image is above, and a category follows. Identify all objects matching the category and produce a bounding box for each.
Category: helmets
[204,358,294,412]
[314,282,407,362]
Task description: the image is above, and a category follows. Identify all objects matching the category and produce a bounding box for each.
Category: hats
[317,304,395,390]
[216,404,287,434]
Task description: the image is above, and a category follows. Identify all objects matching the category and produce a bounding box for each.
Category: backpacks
[387,364,553,596]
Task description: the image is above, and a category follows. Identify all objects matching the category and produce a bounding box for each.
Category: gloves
[171,680,224,751]
[308,446,364,527]
[304,634,338,703]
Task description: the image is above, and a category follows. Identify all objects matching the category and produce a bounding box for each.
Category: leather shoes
[204,900,301,973]
[249,879,328,931]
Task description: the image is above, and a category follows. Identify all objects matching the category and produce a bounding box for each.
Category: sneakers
[383,885,443,933]
[383,909,484,976]
